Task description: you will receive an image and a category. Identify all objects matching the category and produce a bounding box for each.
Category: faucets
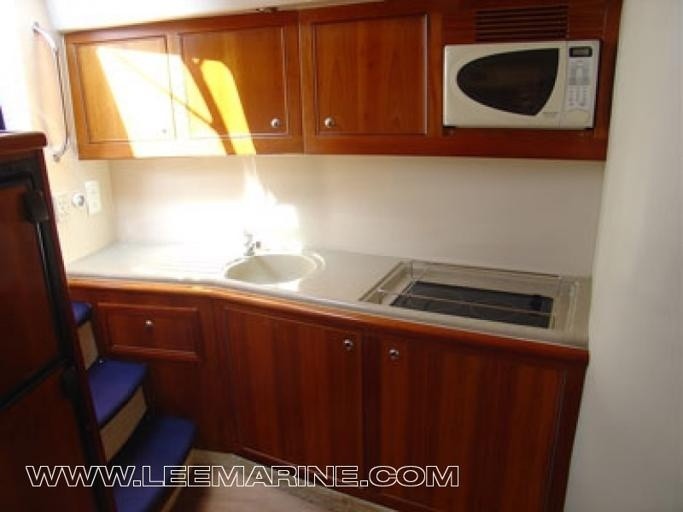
[241,231,257,257]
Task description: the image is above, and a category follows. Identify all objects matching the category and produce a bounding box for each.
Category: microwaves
[442,40,600,130]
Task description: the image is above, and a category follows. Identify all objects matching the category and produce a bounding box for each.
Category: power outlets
[84,179,103,216]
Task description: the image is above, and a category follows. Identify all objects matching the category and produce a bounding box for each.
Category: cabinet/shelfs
[299,0,622,161]
[0,131,116,511]
[213,289,590,512]
[68,278,236,454]
[64,4,300,159]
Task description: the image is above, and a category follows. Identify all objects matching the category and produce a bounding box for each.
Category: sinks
[221,247,325,288]
